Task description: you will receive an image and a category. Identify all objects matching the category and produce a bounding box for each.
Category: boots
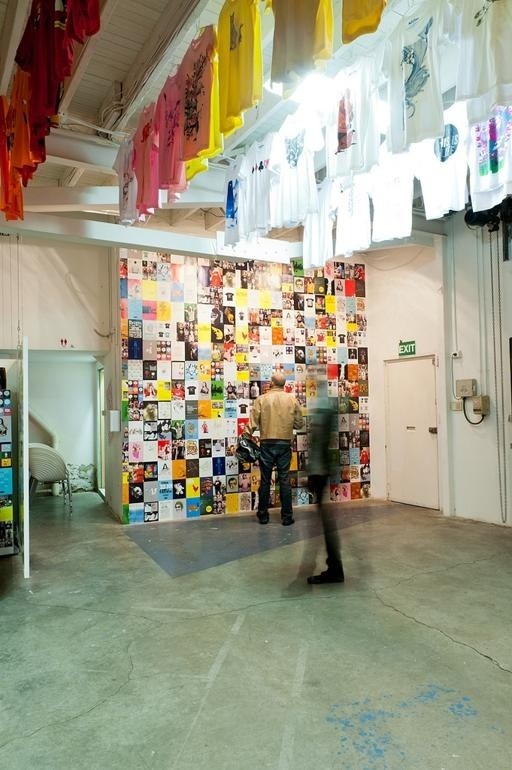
[307,559,345,585]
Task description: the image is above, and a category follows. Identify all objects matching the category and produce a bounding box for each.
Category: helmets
[235,438,260,464]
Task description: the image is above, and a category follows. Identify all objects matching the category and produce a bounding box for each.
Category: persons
[306,407,345,585]
[233,372,305,526]
[119,252,371,521]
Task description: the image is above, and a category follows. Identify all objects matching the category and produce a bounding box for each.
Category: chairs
[29,442,73,506]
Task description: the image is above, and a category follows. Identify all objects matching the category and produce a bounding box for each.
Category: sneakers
[259,516,269,524]
[282,517,294,526]
